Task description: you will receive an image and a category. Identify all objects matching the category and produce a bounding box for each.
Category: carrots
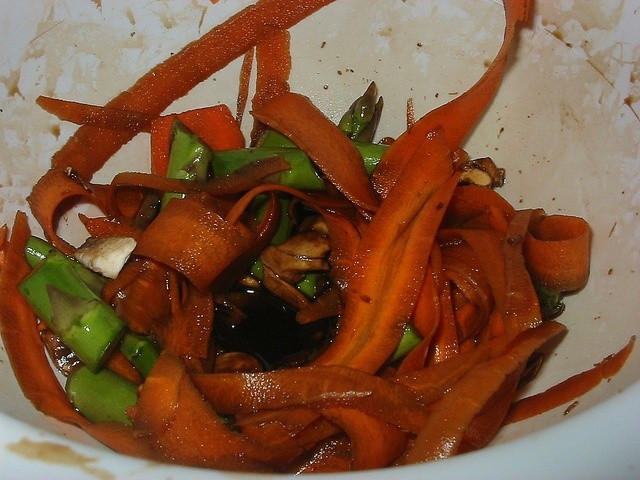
[0,1,636,474]
